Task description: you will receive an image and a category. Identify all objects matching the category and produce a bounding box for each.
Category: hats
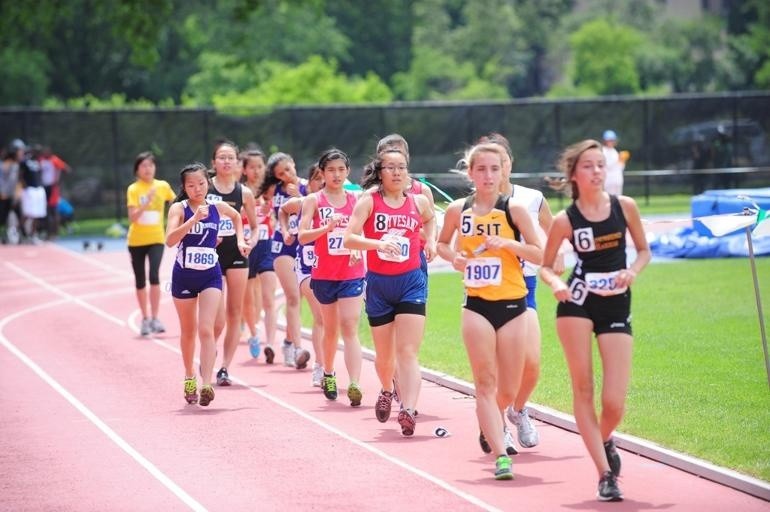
[603,130,619,141]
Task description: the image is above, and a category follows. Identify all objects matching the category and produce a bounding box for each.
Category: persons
[206,139,260,386]
[686,141,704,196]
[437,142,544,480]
[711,125,731,189]
[126,151,177,337]
[0,139,76,245]
[376,133,436,415]
[240,150,276,364]
[343,147,436,436]
[475,134,566,456]
[540,140,652,503]
[165,162,252,406]
[601,130,627,196]
[278,163,327,386]
[256,152,308,370]
[297,149,365,407]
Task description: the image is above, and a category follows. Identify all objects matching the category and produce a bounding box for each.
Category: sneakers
[480,434,497,455]
[506,405,539,448]
[503,429,525,455]
[248,336,260,358]
[597,470,624,502]
[375,389,394,422]
[284,347,296,367]
[264,347,274,364]
[199,384,215,406]
[313,366,324,387]
[603,439,621,477]
[391,380,403,403]
[347,384,362,406]
[217,367,235,385]
[322,376,341,398]
[398,410,416,438]
[140,319,150,336]
[294,349,310,369]
[151,318,166,334]
[494,456,513,480]
[183,376,199,404]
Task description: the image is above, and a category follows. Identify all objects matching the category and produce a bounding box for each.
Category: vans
[649,121,764,183]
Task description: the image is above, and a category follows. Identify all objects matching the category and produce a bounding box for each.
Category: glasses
[381,166,407,170]
